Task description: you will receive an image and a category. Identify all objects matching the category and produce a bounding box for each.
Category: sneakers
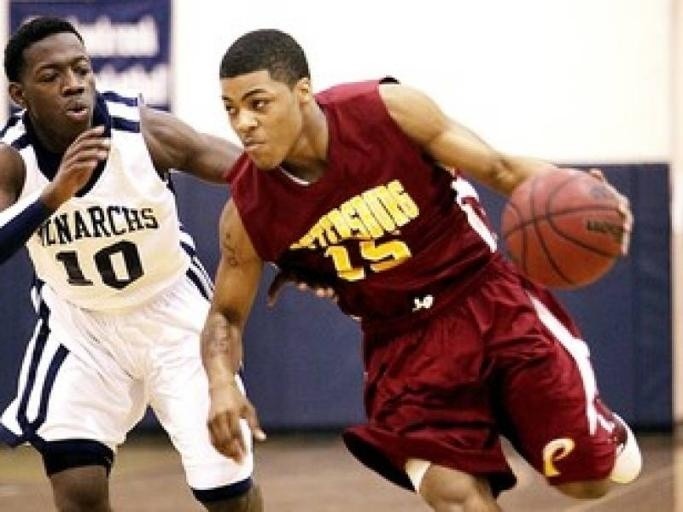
[607,412,643,484]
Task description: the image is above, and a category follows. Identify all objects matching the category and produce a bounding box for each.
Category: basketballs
[501,168,624,290]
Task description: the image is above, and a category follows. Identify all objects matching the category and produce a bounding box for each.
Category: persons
[200,30,642,512]
[0,14,340,512]
